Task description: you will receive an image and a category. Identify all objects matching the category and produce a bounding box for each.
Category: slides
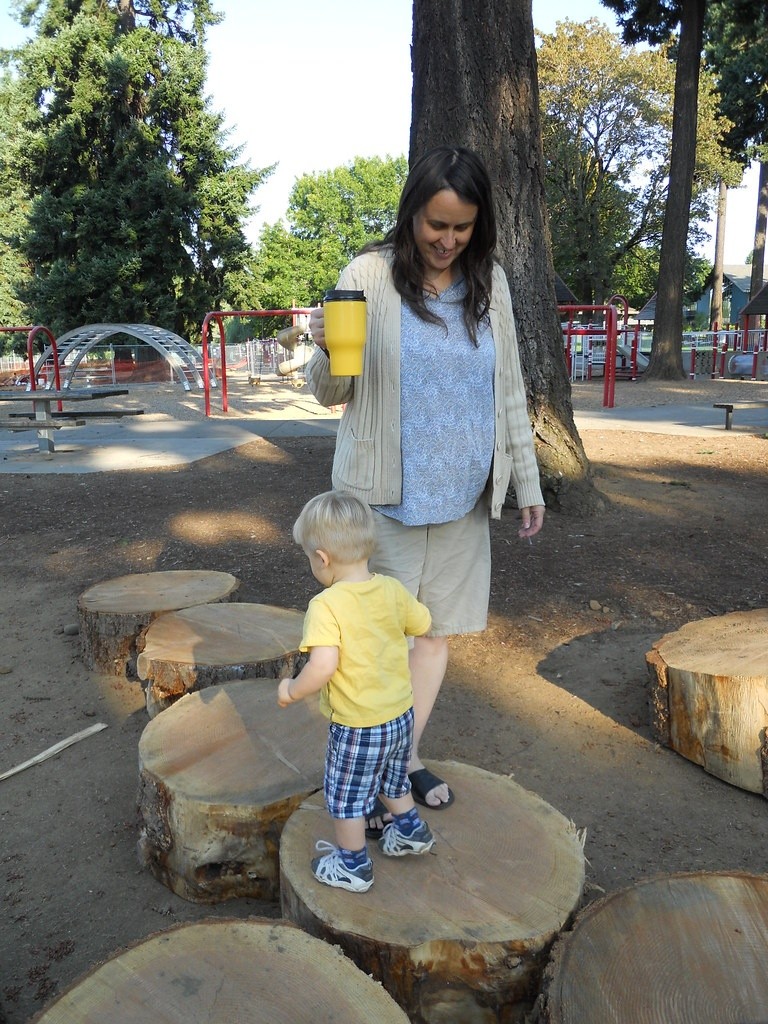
[277,324,315,377]
[617,344,650,369]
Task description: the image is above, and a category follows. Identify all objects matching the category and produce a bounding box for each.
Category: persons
[304,148,544,839]
[279,490,436,892]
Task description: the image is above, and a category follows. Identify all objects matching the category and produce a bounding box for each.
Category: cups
[323,288,367,376]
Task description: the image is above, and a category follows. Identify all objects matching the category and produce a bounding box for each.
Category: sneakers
[377,820,437,856]
[310,839,375,892]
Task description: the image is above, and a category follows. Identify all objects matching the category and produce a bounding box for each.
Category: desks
[72,367,112,388]
[0,389,129,453]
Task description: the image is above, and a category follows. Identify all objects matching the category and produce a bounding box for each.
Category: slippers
[408,766,454,810]
[363,798,395,840]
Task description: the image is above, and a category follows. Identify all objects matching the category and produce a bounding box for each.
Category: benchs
[712,401,768,430]
[0,419,86,427]
[9,410,145,418]
[72,375,113,380]
